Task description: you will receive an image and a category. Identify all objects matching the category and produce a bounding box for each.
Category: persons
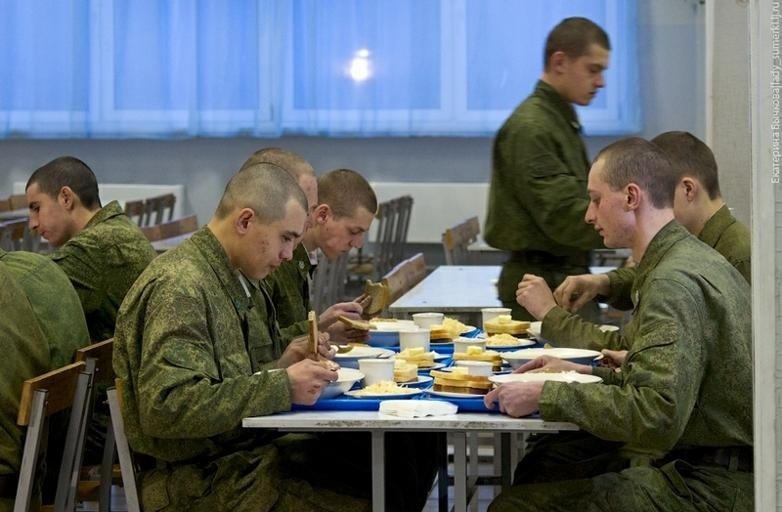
[513,128,752,453]
[482,14,611,329]
[0,157,158,511]
[110,146,437,512]
[484,135,753,510]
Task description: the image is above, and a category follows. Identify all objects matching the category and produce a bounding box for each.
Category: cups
[412,313,445,326]
[399,328,431,350]
[456,359,493,378]
[359,359,396,386]
[482,306,512,327]
[455,337,487,356]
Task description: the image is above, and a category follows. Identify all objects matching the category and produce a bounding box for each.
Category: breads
[453,347,503,371]
[308,311,318,354]
[483,315,530,335]
[338,315,377,330]
[389,346,434,383]
[364,278,388,319]
[429,369,493,396]
[429,325,454,343]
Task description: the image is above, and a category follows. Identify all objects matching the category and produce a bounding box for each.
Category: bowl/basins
[488,373,600,387]
[254,367,364,398]
[333,349,397,368]
[367,319,418,346]
[500,346,600,368]
[526,321,551,345]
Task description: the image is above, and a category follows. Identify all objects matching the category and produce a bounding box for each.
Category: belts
[504,249,592,266]
[670,445,753,473]
[174,433,252,466]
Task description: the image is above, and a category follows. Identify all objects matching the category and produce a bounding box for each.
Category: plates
[423,387,485,400]
[486,338,535,349]
[344,388,420,398]
[398,375,434,385]
